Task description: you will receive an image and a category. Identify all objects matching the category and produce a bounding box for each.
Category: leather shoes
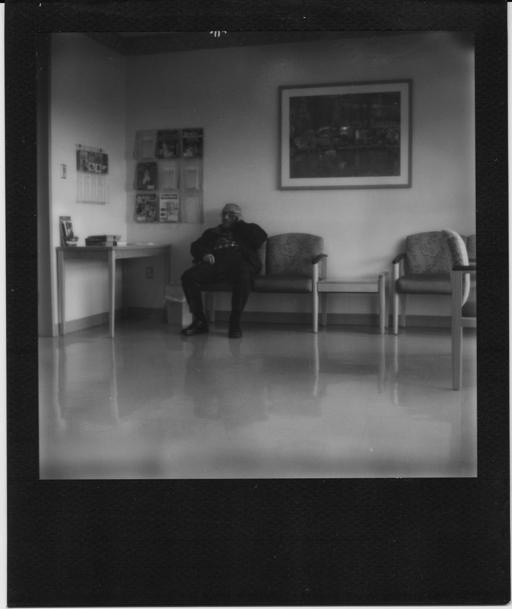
[227,317,248,340]
[180,319,209,336]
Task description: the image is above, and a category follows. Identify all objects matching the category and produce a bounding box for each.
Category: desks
[56,239,173,341]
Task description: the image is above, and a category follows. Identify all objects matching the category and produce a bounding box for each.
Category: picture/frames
[277,78,412,190]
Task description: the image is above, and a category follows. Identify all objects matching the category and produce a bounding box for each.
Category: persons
[181,203,268,338]
[289,123,400,176]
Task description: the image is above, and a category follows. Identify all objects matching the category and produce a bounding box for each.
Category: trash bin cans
[166,280,193,330]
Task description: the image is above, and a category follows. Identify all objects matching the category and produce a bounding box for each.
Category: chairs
[186,231,327,336]
[386,228,476,391]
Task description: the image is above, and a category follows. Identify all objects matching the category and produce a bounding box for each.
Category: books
[132,128,203,225]
